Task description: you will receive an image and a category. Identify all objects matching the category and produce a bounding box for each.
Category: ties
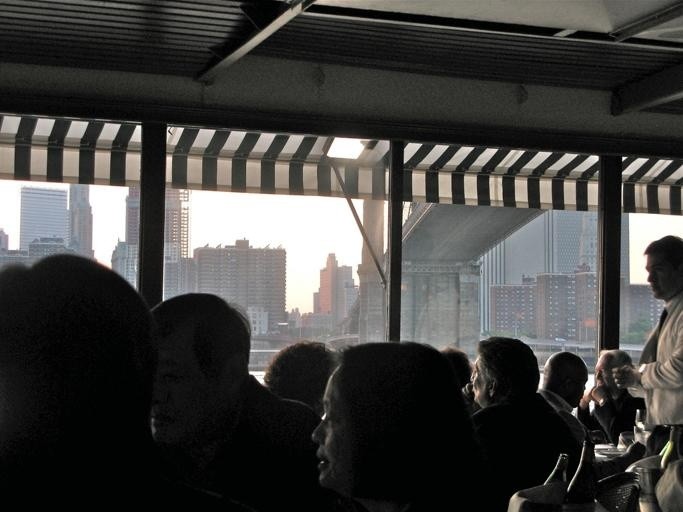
[639,309,668,364]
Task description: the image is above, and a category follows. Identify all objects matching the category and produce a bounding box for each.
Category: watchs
[638,363,646,374]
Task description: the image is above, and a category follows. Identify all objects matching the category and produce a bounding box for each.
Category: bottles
[539,452,573,509]
[566,439,602,510]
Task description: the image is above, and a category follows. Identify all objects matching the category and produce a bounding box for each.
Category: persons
[313,342,505,512]
[150,293,342,511]
[0,254,246,510]
[612,236,681,510]
[442,336,647,512]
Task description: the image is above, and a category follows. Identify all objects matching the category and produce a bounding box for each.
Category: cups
[632,407,649,437]
